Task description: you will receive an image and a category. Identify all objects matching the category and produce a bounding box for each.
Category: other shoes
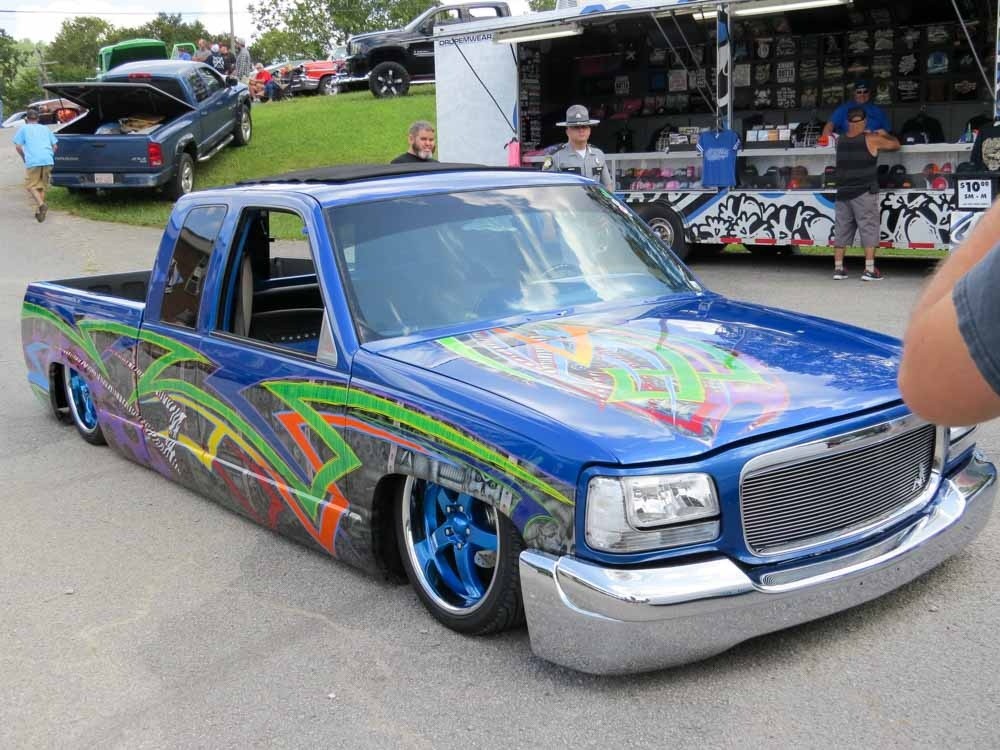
[36,204,48,223]
[253,92,273,103]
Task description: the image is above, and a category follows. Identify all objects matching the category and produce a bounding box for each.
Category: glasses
[856,89,869,94]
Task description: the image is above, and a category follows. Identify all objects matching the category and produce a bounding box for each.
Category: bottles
[966,122,972,143]
[960,128,967,143]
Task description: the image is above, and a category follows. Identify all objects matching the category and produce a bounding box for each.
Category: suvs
[327,1,514,100]
[43,59,257,205]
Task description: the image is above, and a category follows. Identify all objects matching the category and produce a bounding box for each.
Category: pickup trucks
[18,160,998,679]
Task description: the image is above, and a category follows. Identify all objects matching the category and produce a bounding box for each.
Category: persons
[963,93,993,134]
[179,39,236,78]
[391,120,442,163]
[541,103,616,195]
[896,191,1000,426]
[833,108,900,280]
[10,108,58,223]
[821,79,893,140]
[248,62,294,103]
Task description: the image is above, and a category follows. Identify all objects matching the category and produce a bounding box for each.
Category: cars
[252,44,352,95]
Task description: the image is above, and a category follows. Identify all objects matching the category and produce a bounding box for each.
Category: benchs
[248,279,377,343]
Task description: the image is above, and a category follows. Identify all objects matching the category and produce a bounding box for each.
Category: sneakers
[833,268,848,279]
[861,268,884,280]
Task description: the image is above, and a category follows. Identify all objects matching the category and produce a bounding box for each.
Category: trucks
[431,0,999,266]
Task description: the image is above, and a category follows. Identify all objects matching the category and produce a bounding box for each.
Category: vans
[85,37,196,81]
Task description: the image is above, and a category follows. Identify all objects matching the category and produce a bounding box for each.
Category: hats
[234,36,246,46]
[854,81,868,88]
[556,105,600,126]
[27,109,40,120]
[847,108,866,122]
[211,44,220,53]
